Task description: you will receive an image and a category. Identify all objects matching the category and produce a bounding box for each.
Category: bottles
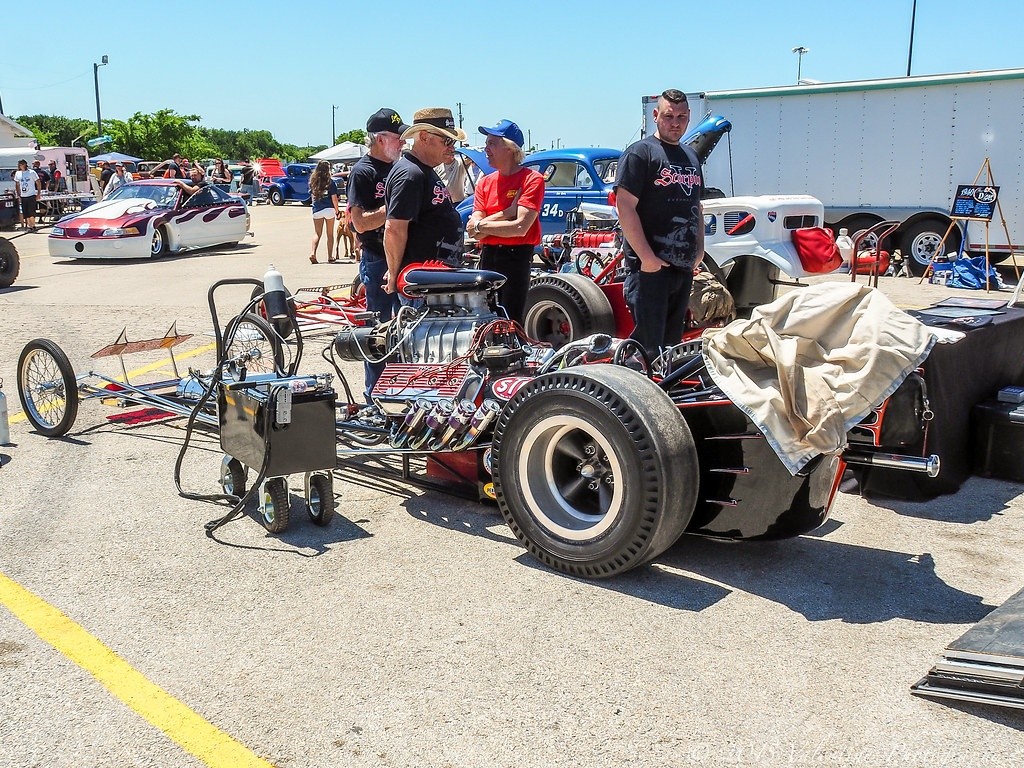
[929,266,934,283]
[835,228,855,268]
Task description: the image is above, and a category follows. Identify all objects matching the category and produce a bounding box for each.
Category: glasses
[420,130,456,147]
[215,163,222,164]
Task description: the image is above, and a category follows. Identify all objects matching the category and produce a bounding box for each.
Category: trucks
[0,146,91,205]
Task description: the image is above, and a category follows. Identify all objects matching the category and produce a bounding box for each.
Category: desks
[36,195,99,225]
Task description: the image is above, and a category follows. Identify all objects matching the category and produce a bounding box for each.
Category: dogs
[336,211,355,260]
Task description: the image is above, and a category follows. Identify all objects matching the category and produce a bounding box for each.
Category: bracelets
[473,220,480,233]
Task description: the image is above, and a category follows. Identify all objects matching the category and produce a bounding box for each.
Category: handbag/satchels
[164,169,170,178]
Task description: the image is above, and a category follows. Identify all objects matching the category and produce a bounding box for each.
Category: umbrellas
[90,152,143,163]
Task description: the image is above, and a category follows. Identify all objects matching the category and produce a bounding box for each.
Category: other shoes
[328,257,336,262]
[309,257,318,264]
[248,204,251,206]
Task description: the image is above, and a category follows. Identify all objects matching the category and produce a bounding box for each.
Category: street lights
[332,104,339,146]
[93,54,108,155]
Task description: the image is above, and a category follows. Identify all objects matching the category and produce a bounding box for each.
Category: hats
[478,119,524,148]
[97,161,103,164]
[366,108,409,135]
[48,159,56,165]
[399,107,468,141]
[116,161,124,167]
[172,154,184,159]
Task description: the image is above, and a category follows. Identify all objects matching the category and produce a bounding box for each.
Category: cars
[453,109,737,291]
[47,178,250,260]
[109,158,352,207]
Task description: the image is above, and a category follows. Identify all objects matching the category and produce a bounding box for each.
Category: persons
[15,159,41,234]
[192,157,206,181]
[380,108,464,312]
[168,153,190,180]
[614,89,704,361]
[99,162,115,195]
[211,158,234,199]
[238,157,254,206]
[173,168,213,203]
[345,108,411,394]
[432,146,483,209]
[46,160,61,221]
[466,118,544,334]
[308,159,338,264]
[30,160,50,223]
[103,161,133,198]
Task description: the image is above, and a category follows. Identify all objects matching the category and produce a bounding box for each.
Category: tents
[309,139,369,161]
